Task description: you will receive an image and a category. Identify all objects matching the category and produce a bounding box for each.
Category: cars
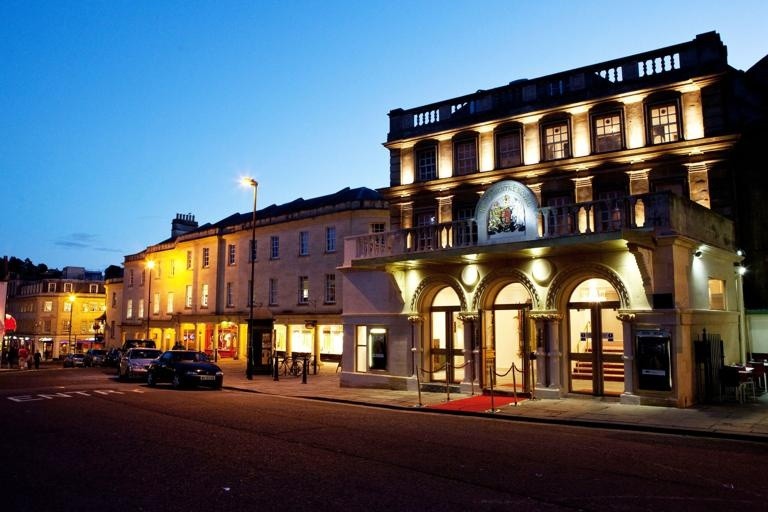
[146,349,224,390]
[63,339,163,381]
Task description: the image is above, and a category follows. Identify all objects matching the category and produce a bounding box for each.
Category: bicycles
[274,352,322,377]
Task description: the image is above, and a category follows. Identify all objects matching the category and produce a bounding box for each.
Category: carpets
[500,382,522,388]
[425,394,527,413]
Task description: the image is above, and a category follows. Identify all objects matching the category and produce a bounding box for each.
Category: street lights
[244,177,259,382]
[68,296,75,350]
[145,260,155,340]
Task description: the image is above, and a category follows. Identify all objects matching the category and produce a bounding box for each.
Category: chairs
[723,358,768,406]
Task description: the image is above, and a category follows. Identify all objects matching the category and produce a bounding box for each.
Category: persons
[8,345,42,370]
[172,341,184,350]
